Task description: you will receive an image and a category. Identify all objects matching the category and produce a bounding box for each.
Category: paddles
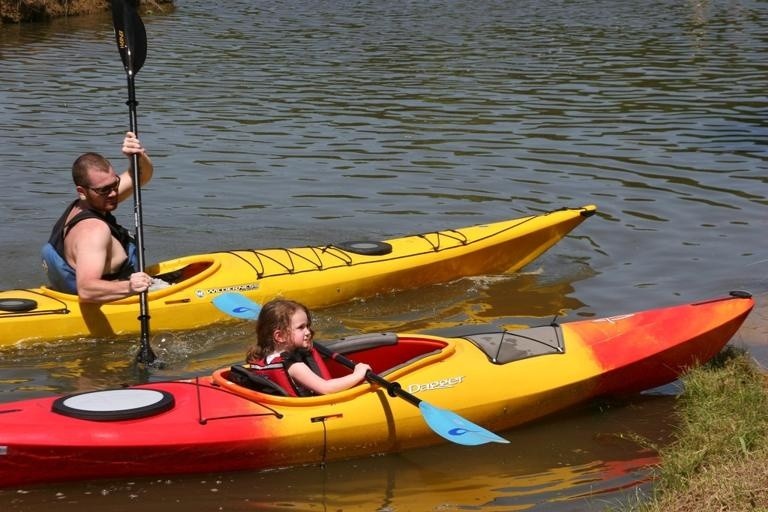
[110,1,158,363]
[210,293,511,446]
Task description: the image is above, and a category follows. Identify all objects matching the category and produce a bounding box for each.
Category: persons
[248,297,371,401]
[40,128,172,308]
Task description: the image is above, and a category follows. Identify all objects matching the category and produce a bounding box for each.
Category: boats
[1,196,597,359]
[0,293,755,472]
[1,399,740,512]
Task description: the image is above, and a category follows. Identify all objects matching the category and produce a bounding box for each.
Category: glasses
[87,175,120,195]
